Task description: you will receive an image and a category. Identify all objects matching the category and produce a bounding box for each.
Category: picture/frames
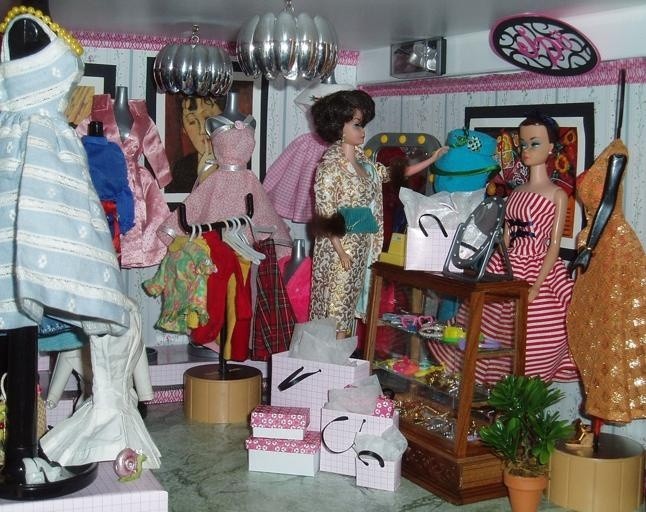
[146,57,269,212]
[464,102,594,261]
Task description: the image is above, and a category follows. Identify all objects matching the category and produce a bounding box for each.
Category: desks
[0,398,168,512]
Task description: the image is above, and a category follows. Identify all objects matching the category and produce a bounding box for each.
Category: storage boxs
[245,404,320,477]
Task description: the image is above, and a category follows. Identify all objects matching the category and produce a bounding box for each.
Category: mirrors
[363,132,442,253]
[442,195,513,283]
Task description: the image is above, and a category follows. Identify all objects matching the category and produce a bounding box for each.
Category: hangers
[185,214,266,265]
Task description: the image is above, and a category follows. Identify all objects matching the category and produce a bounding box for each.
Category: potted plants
[479,373,576,512]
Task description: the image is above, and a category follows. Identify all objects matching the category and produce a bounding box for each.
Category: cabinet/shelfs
[363,261,532,506]
